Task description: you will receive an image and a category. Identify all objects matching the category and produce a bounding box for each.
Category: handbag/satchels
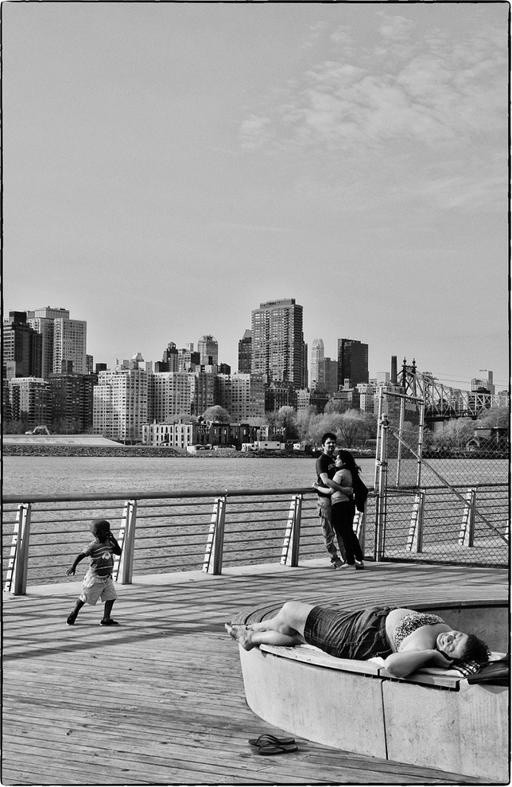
[467,651,510,689]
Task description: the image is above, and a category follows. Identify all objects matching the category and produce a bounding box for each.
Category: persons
[311,448,367,570]
[221,597,490,681]
[314,430,359,570]
[65,518,126,628]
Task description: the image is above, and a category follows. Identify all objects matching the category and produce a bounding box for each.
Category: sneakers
[66,612,78,625]
[329,559,365,571]
[100,619,119,626]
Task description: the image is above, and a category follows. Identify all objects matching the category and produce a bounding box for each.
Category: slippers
[246,734,295,746]
[250,744,299,756]
